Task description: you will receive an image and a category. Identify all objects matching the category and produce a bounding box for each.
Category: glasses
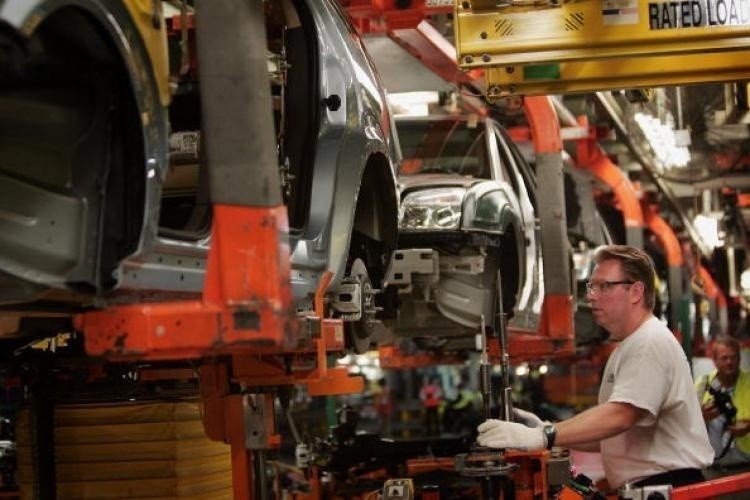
[586,280,635,291]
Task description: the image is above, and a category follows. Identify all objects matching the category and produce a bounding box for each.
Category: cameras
[706,386,738,420]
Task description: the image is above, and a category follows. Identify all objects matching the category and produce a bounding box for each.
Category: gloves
[477,420,547,451]
[511,407,552,427]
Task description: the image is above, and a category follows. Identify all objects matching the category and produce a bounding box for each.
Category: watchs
[544,426,557,451]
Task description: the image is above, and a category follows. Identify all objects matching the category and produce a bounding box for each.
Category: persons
[477,244,716,500]
[376,366,542,443]
[693,336,750,500]
